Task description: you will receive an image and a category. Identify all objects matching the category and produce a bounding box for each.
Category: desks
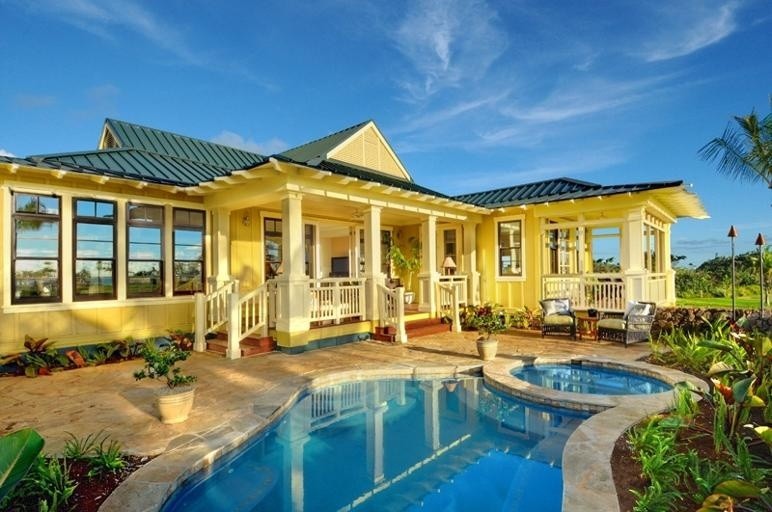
[577,315,599,340]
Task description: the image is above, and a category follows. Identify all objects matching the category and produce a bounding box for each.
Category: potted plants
[385,234,422,305]
[130,332,199,423]
[497,302,542,329]
[462,305,509,361]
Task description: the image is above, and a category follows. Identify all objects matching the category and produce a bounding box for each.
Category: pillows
[543,300,568,314]
[627,305,650,322]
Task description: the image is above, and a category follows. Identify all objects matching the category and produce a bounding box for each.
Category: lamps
[441,256,458,277]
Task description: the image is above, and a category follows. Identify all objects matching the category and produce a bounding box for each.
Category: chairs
[596,302,655,349]
[541,297,573,338]
